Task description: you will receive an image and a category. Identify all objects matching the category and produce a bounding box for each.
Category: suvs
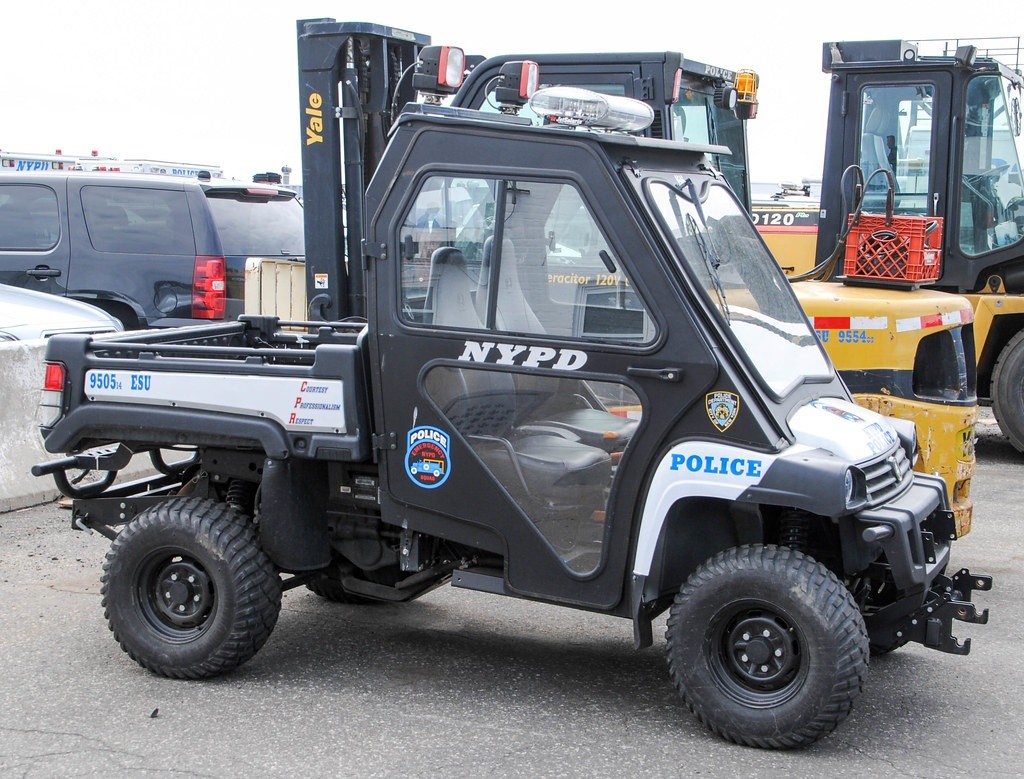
[1,170,306,330]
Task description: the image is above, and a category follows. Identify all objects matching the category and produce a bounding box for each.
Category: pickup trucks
[31,45,997,750]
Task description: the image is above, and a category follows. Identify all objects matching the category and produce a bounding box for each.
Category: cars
[2,283,127,345]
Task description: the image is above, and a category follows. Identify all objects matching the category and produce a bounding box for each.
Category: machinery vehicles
[294,17,1023,539]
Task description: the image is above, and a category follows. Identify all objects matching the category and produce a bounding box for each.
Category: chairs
[424,235,640,518]
[858,108,928,214]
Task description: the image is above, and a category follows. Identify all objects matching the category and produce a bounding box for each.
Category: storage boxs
[844,213,943,281]
